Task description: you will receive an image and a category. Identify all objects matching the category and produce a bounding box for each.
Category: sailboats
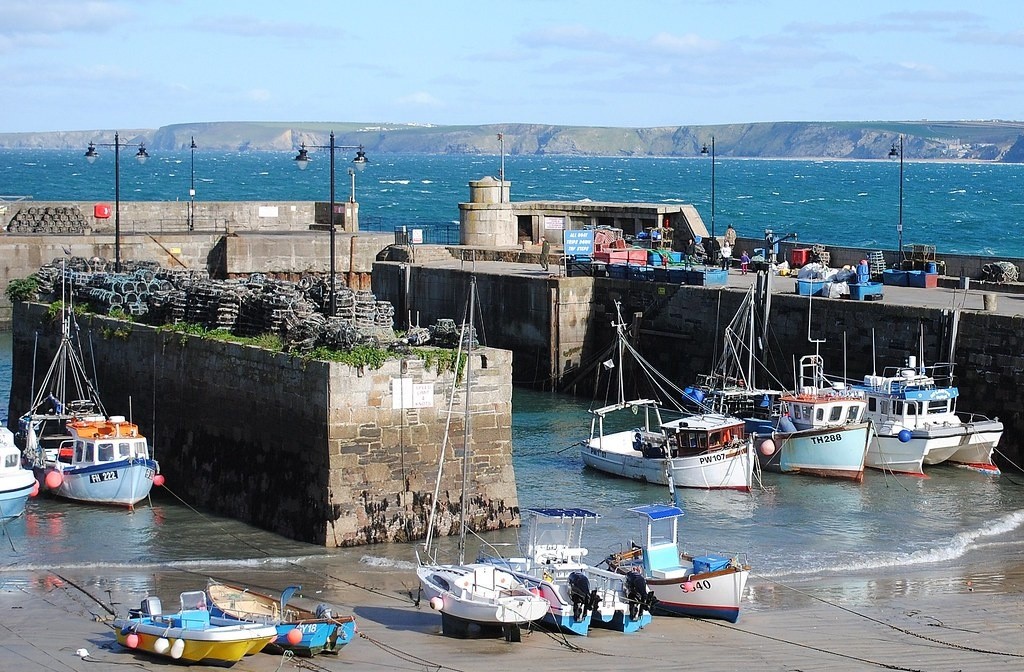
[415,275,551,642]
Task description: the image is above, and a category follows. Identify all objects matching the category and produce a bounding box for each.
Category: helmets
[728,223,734,227]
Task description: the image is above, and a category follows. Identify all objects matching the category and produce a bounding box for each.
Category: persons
[720,241,731,275]
[739,250,750,275]
[724,223,737,249]
[684,238,695,267]
[540,236,550,272]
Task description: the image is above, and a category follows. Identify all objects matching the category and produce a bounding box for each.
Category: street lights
[292,128,371,317]
[700,137,715,258]
[888,135,903,270]
[191,135,198,231]
[84,131,151,273]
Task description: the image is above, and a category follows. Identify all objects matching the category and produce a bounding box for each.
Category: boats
[13,258,160,506]
[606,462,752,623]
[679,273,1004,484]
[0,420,36,518]
[475,498,660,636]
[204,578,357,658]
[581,298,753,492]
[112,575,278,668]
